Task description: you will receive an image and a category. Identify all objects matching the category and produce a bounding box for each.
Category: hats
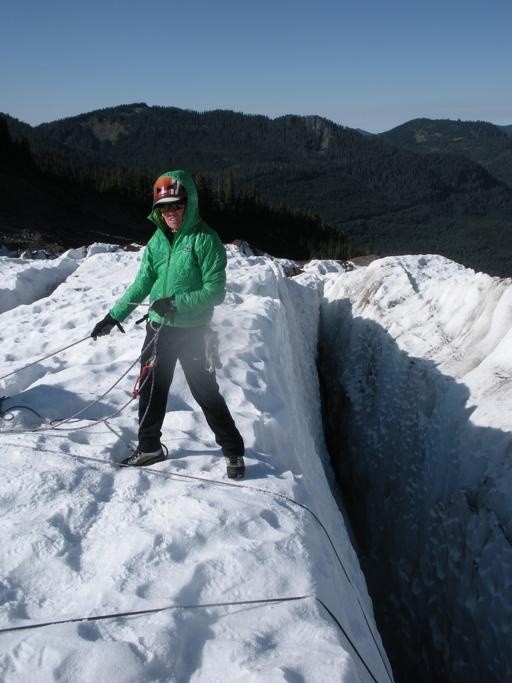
[151,175,188,208]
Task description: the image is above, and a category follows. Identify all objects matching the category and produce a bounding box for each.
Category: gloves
[91,312,118,341]
[150,293,177,318]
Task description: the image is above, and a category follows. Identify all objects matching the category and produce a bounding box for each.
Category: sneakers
[224,453,246,479]
[119,447,166,467]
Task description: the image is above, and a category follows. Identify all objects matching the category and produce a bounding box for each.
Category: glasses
[157,204,186,213]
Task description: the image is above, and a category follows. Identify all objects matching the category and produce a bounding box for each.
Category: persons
[90,166,250,481]
